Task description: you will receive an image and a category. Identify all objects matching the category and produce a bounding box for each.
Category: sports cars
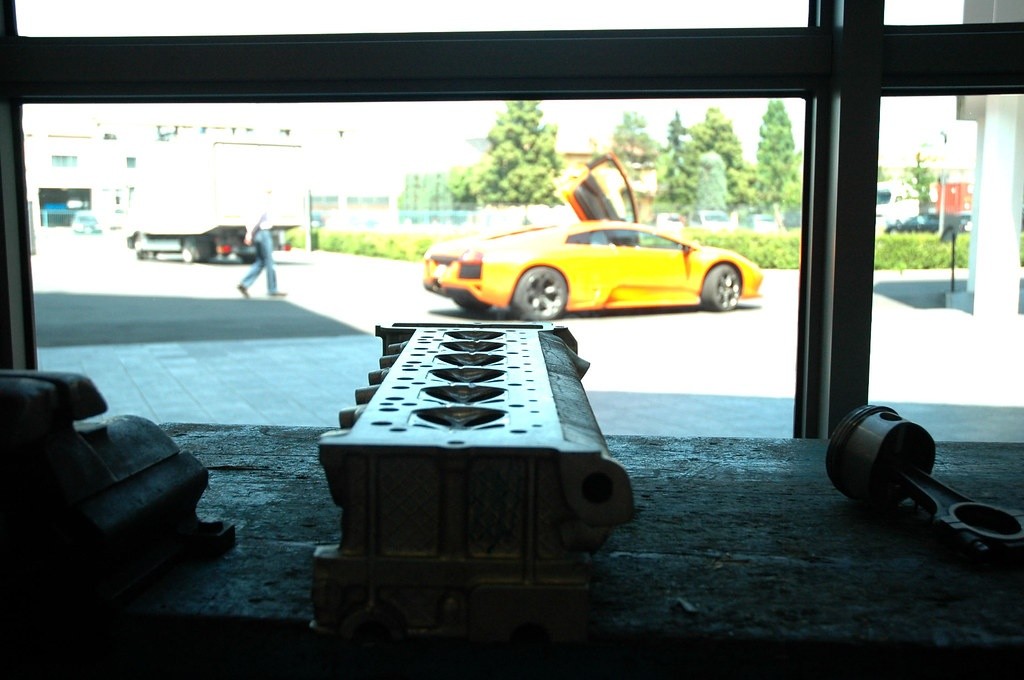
[422,148,766,321]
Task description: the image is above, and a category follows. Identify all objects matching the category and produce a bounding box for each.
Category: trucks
[877,173,972,234]
[117,122,311,264]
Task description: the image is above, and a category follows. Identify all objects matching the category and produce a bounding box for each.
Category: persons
[237,188,287,297]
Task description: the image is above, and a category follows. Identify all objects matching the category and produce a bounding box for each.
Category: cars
[71,211,101,234]
[886,213,960,235]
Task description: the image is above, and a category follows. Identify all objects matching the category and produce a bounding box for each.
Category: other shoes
[268,291,287,297]
[238,285,249,298]
